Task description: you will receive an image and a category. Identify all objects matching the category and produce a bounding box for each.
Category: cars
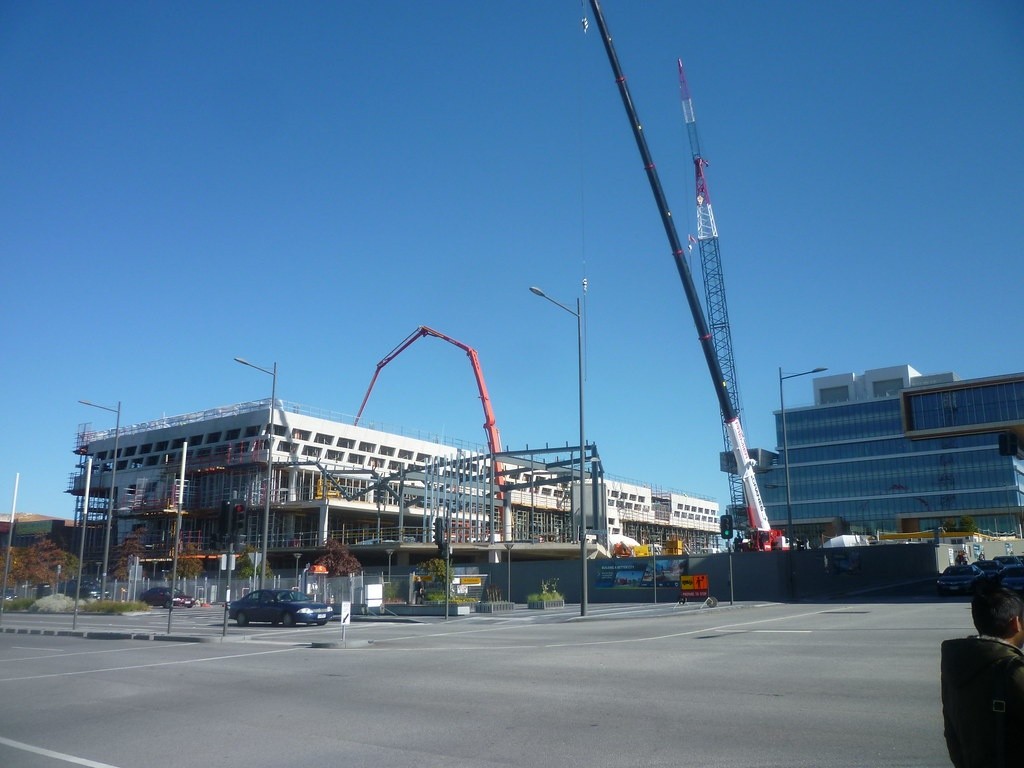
[0,579,195,608]
[228,589,334,627]
[999,564,1024,592]
[993,556,1024,565]
[972,559,1003,581]
[936,565,985,596]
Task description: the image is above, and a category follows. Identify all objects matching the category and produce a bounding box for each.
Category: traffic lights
[433,517,444,545]
[721,515,733,539]
[227,499,246,534]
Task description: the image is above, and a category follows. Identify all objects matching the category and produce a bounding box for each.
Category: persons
[941,568,1024,768]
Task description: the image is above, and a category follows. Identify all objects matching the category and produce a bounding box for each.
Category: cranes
[677,57,752,538]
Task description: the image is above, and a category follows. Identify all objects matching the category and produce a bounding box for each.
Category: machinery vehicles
[581,0,810,552]
[354,325,514,542]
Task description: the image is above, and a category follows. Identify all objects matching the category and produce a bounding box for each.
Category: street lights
[386,549,394,582]
[78,399,121,602]
[649,535,660,605]
[779,367,828,552]
[531,287,587,617]
[236,357,277,590]
[294,553,302,591]
[505,544,515,602]
